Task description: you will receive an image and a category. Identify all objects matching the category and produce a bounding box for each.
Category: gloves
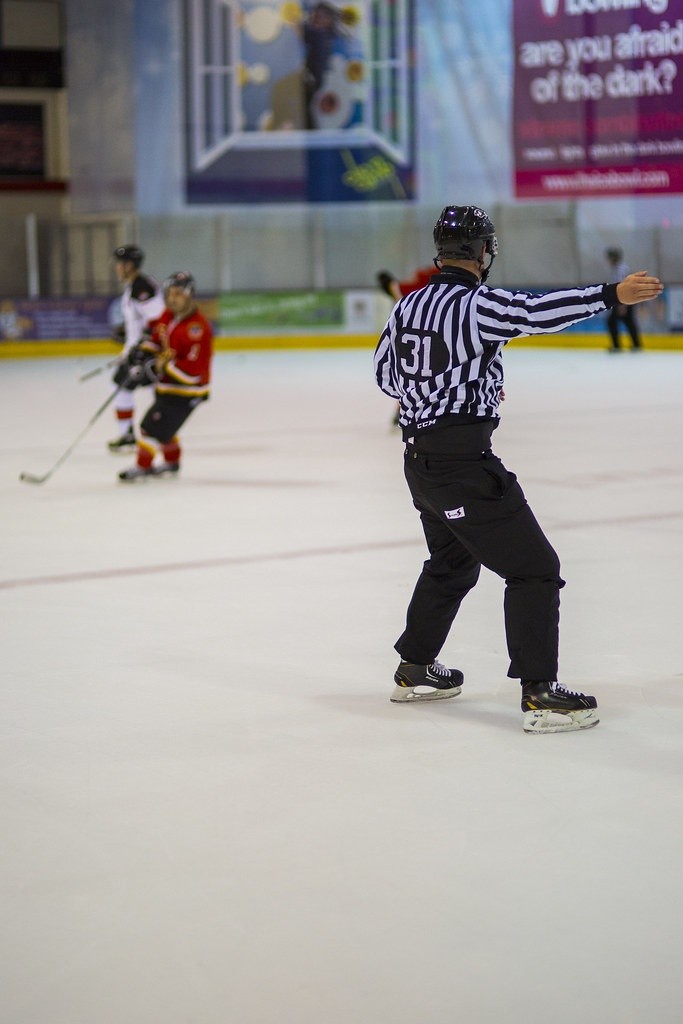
[137,352,165,387]
[114,349,153,389]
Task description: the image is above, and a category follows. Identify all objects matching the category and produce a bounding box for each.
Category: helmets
[111,245,143,282]
[433,204,499,260]
[161,271,196,311]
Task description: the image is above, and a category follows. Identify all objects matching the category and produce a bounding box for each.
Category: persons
[298,6,343,131]
[116,272,214,480]
[374,204,664,733]
[379,266,438,302]
[109,246,186,450]
[604,246,644,351]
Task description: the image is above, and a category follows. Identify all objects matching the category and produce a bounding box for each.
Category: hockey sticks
[20,375,129,485]
[78,354,121,380]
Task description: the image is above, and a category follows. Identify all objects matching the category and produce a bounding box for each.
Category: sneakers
[106,427,138,453]
[520,680,601,733]
[152,461,179,479]
[391,655,465,704]
[118,466,154,485]
